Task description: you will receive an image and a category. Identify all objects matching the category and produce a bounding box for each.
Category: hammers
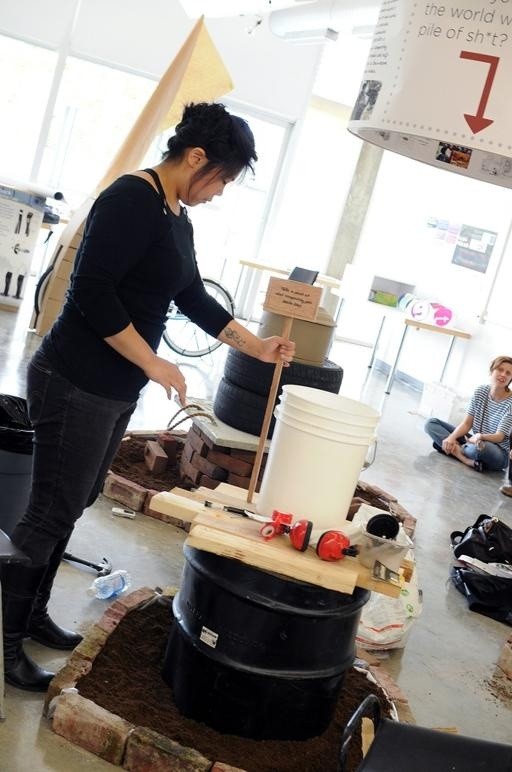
[62,550,112,577]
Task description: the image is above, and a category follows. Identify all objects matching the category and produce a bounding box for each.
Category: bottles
[85,569,132,601]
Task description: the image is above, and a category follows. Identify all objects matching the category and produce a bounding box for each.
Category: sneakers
[500,483,512,497]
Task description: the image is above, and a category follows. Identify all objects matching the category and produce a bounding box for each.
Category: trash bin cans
[0,393,35,538]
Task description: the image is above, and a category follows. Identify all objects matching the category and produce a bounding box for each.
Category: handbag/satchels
[451,566,512,627]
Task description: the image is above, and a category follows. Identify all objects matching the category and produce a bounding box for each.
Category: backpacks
[449,513,512,566]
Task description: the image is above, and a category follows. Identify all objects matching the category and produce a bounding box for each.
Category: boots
[1,538,83,692]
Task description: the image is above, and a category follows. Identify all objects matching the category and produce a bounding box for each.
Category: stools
[330,694,512,770]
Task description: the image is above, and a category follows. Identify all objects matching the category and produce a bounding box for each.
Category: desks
[328,286,472,400]
[228,258,342,328]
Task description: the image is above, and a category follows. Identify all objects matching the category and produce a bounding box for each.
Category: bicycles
[34,263,237,358]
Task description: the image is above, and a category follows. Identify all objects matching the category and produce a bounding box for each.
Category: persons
[425,356,512,473]
[0,103,296,692]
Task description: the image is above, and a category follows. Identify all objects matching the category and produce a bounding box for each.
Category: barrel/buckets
[163,542,371,741]
[256,384,381,529]
